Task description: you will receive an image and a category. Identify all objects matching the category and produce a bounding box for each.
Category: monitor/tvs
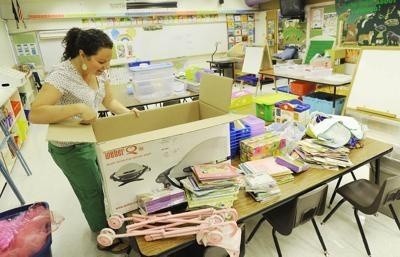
[280,0,305,19]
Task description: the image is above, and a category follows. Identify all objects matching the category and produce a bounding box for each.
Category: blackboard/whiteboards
[82,11,228,113]
[345,46,400,128]
[241,46,264,74]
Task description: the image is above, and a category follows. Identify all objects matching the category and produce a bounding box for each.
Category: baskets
[230,121,252,160]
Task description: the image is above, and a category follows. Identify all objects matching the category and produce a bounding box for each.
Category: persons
[27,27,140,233]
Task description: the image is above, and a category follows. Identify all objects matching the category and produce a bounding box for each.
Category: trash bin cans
[0,202,52,257]
[370,143,400,220]
[301,91,347,115]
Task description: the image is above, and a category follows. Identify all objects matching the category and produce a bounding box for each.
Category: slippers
[97,238,129,254]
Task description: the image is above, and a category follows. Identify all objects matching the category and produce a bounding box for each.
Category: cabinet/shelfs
[0,72,35,168]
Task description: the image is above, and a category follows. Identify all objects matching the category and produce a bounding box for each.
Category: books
[134,154,311,216]
